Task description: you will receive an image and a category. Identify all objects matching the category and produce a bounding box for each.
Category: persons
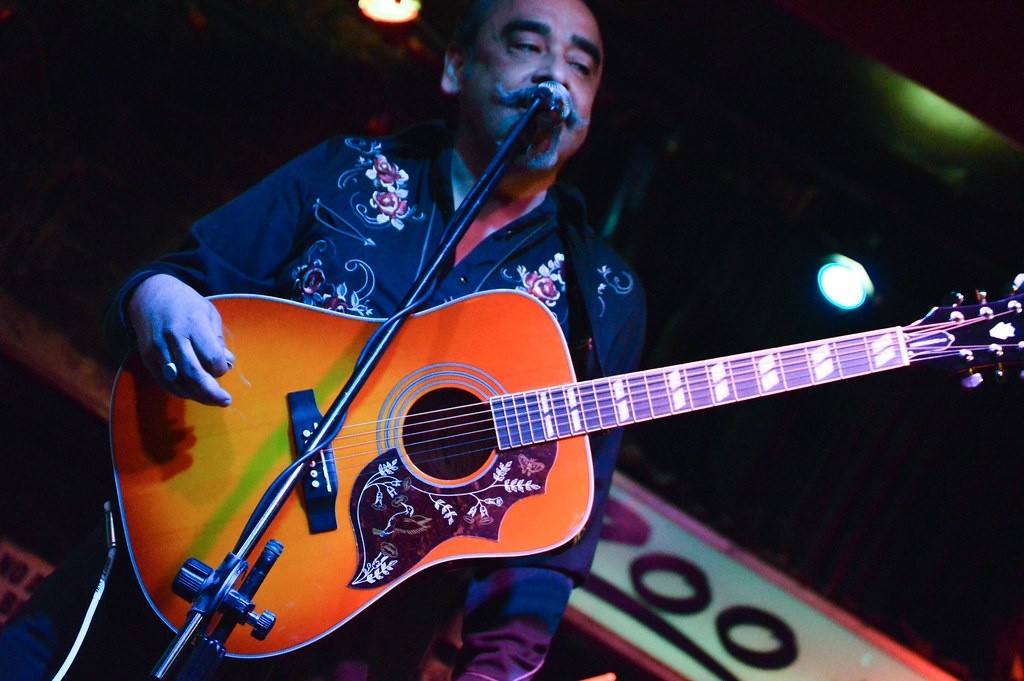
[2,0,645,681]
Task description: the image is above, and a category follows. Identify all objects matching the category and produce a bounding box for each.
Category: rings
[163,362,184,383]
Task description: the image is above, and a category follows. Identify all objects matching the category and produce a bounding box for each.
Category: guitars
[106,268,1024,660]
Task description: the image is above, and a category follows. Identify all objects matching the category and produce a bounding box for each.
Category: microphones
[527,81,574,126]
